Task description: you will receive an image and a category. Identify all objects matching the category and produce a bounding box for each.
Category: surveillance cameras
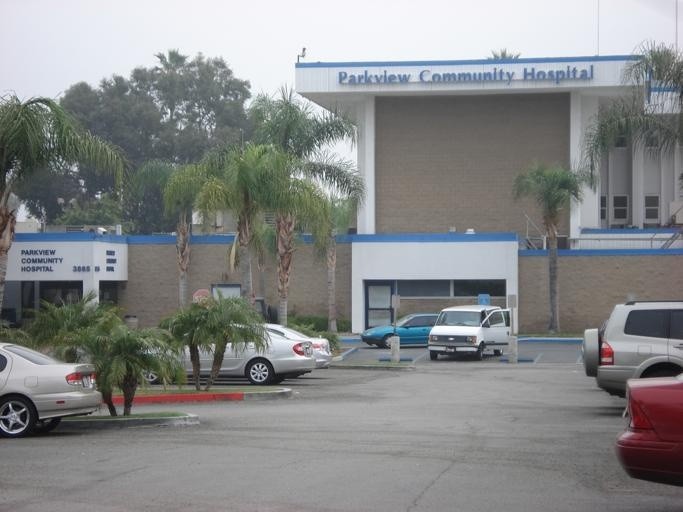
[97,227,107,237]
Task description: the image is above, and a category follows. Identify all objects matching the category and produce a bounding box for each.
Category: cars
[137,322,332,385]
[613,372,682,487]
[359,313,439,349]
[0,342,103,438]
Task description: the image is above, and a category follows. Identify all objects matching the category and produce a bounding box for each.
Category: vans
[427,304,511,361]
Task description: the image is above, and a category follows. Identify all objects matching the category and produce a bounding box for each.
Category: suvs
[580,299,682,399]
